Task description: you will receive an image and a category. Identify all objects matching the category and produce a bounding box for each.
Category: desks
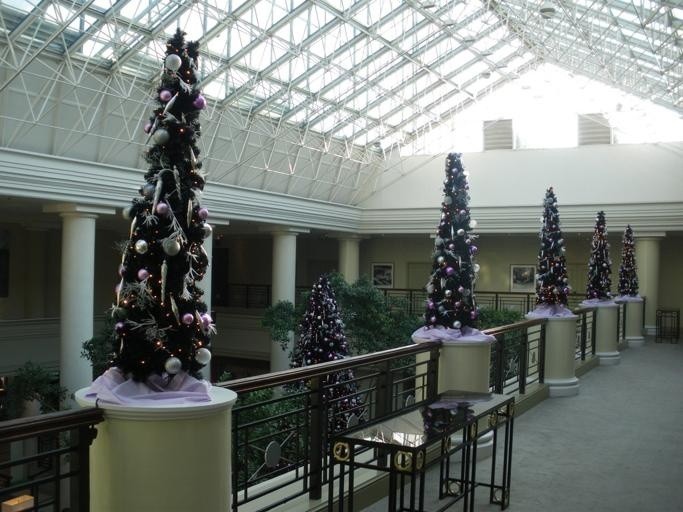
[328,390,516,512]
[655,308,680,344]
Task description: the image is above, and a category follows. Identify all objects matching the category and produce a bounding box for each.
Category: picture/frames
[371,263,393,289]
[510,264,537,293]
